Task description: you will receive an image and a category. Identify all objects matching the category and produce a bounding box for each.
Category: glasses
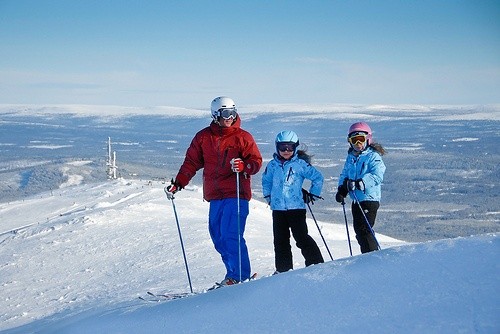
[277,143,296,152]
[347,134,367,145]
[217,108,237,119]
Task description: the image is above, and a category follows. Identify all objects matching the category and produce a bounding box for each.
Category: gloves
[343,177,365,192]
[164,182,181,200]
[302,188,313,203]
[336,185,349,202]
[229,158,247,174]
[263,195,271,205]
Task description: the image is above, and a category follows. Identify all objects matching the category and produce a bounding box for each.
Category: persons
[261,131,324,276]
[164,97,262,286]
[335,122,386,254]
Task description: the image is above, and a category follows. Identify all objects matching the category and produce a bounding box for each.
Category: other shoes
[219,277,249,288]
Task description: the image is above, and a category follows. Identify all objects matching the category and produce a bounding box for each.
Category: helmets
[209,96,237,120]
[274,130,299,145]
[347,121,372,146]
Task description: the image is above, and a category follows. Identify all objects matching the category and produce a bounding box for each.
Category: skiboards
[138,272,258,303]
